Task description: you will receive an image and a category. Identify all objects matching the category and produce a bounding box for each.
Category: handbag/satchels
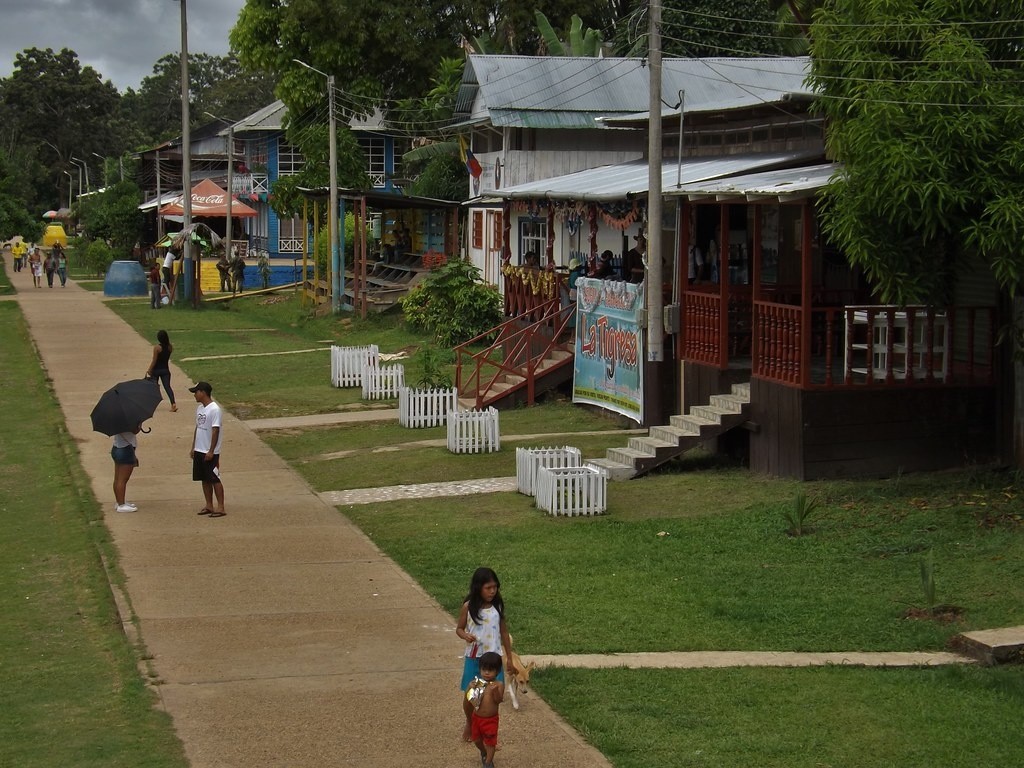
[133,456,139,467]
[144,372,157,384]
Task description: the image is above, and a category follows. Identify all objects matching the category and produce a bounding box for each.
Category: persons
[20,240,34,267]
[217,252,232,292]
[111,423,142,513]
[188,381,227,518]
[229,250,246,293]
[627,228,646,284]
[162,249,181,289]
[53,240,63,252]
[12,242,23,272]
[525,251,536,265]
[687,233,704,284]
[29,249,42,288]
[456,567,516,742]
[464,651,504,768]
[385,227,412,264]
[43,252,69,288]
[597,250,613,270]
[569,259,583,288]
[150,263,162,309]
[148,330,178,413]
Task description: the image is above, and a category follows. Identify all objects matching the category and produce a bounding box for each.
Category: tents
[159,178,259,261]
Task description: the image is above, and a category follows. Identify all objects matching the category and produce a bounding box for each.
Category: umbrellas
[89,379,164,438]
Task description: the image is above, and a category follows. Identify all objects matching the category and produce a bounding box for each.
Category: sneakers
[114,502,134,510]
[116,505,137,513]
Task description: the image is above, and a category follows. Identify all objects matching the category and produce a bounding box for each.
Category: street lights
[64,171,71,209]
[72,157,89,192]
[203,112,231,262]
[293,59,339,316]
[92,153,107,186]
[69,161,81,196]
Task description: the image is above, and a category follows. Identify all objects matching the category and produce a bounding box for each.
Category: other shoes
[482,754,494,768]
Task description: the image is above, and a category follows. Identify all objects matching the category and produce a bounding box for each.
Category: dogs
[500,633,537,710]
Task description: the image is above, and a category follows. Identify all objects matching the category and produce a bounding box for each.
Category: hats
[220,252,225,257]
[234,250,239,255]
[189,382,212,393]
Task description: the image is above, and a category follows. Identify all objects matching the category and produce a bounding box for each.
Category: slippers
[197,509,214,515]
[170,408,178,412]
[209,512,226,517]
